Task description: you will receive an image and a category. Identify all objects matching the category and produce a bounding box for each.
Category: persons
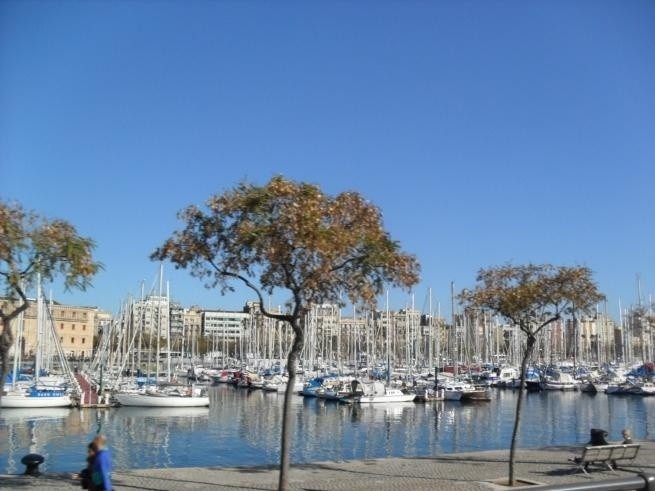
[610,428,634,470]
[573,428,610,469]
[75,442,96,491]
[89,433,115,491]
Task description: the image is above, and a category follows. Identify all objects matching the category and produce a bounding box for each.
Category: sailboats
[0,259,211,410]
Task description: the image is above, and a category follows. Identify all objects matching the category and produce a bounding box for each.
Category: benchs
[567,444,641,479]
